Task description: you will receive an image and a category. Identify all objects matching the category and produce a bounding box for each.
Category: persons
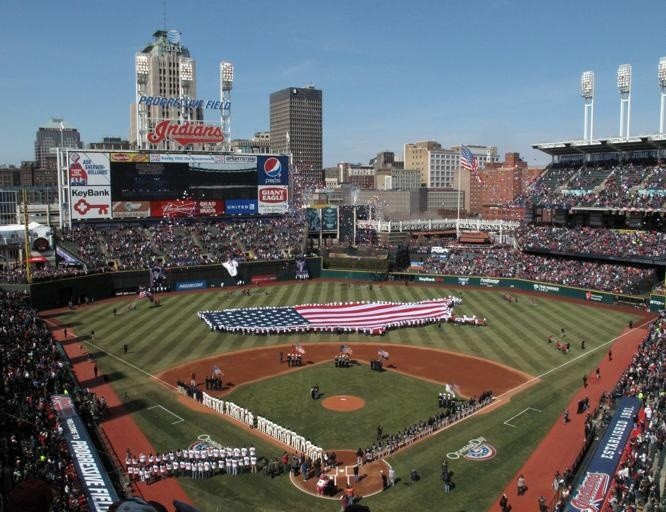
[0,217,308,512]
[309,156,666,511]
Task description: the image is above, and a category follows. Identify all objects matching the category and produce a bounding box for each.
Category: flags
[458,144,483,185]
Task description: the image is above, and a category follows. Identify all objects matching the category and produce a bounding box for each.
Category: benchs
[53,217,309,269]
[509,161,666,214]
